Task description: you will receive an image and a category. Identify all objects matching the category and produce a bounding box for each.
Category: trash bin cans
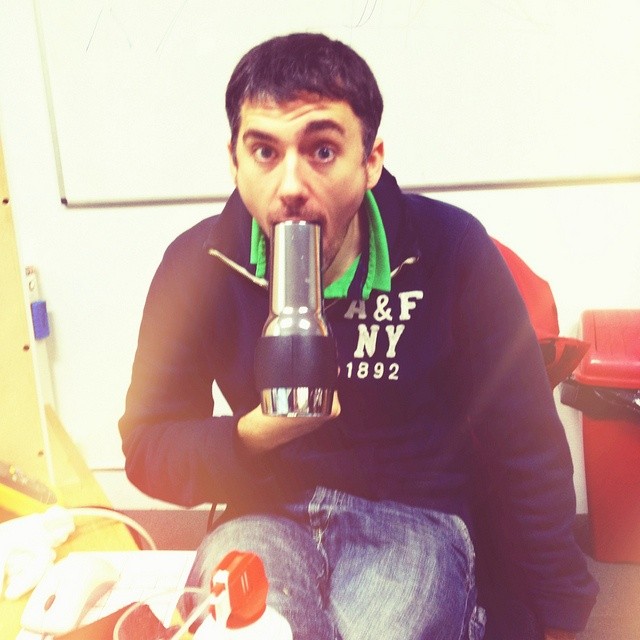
[562,309,640,563]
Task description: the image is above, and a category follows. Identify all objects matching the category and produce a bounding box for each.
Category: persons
[118,33,600,639]
